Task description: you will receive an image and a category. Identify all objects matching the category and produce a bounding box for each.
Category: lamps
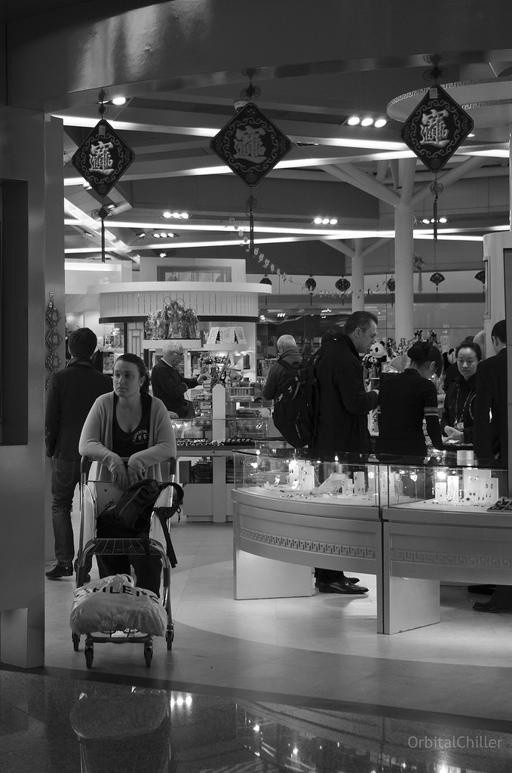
[385,61,512,130]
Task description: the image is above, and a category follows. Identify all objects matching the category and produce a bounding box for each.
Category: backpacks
[272,337,344,449]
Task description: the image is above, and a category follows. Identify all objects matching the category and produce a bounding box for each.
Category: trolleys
[66,458,184,668]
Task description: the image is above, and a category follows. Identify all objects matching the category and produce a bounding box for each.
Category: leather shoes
[473,601,511,613]
[468,585,495,596]
[315,578,369,594]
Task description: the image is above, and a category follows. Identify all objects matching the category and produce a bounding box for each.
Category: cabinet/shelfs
[171,419,268,524]
[232,446,384,634]
[208,321,257,382]
[380,447,512,636]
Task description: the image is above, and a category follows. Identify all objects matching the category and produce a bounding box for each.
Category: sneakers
[46,565,73,579]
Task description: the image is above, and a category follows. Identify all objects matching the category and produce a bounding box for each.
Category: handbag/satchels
[96,479,161,538]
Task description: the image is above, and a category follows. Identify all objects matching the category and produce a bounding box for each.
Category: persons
[442,331,486,389]
[363,340,388,377]
[308,312,381,596]
[78,354,178,594]
[441,342,490,469]
[375,340,454,499]
[46,327,114,579]
[471,320,508,468]
[150,344,211,484]
[263,334,309,403]
